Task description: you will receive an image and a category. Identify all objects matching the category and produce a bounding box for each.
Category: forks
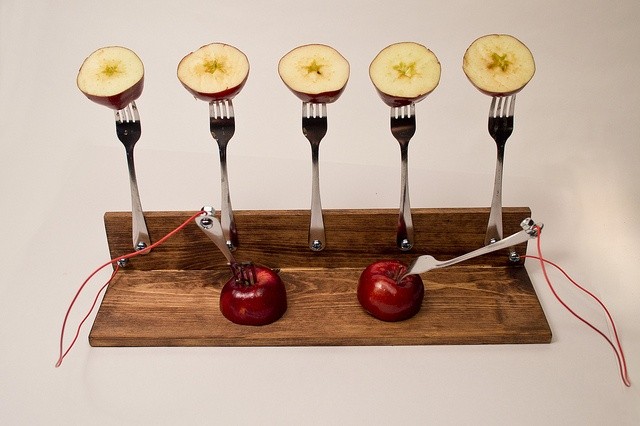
[485,93,514,245]
[195,215,256,288]
[113,98,151,255]
[300,102,328,252]
[209,97,237,252]
[392,224,544,285]
[389,102,415,251]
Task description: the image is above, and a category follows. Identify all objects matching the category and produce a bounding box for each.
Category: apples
[75,42,148,112]
[355,256,428,323]
[175,38,252,100]
[278,40,352,103]
[461,31,538,97]
[218,261,292,328]
[366,36,444,104]
